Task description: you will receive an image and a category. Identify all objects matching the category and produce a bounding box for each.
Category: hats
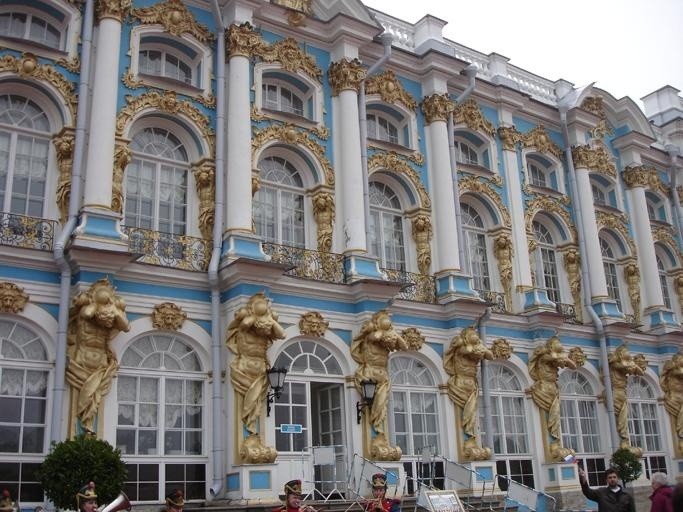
[165,491,184,508]
[76,481,97,510]
[285,480,301,495]
[372,473,387,488]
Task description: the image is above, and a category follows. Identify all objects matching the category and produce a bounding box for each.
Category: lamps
[353,374,377,425]
[263,364,288,419]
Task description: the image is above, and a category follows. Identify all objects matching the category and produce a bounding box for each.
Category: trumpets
[295,503,323,512]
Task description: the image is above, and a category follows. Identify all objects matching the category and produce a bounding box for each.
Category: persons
[671,472,683,512]
[164,489,185,512]
[350,311,408,432]
[624,264,641,312]
[359,473,401,512]
[577,466,636,512]
[411,215,433,275]
[310,193,335,252]
[224,291,286,437]
[51,135,74,226]
[444,325,493,438]
[74,481,99,512]
[659,350,683,441]
[492,235,513,293]
[194,167,215,242]
[528,336,579,439]
[112,146,131,213]
[649,471,674,512]
[64,278,131,439]
[0,488,20,512]
[564,250,582,303]
[272,479,322,512]
[597,346,645,439]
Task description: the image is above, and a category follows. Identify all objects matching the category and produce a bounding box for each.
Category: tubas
[93,490,131,512]
